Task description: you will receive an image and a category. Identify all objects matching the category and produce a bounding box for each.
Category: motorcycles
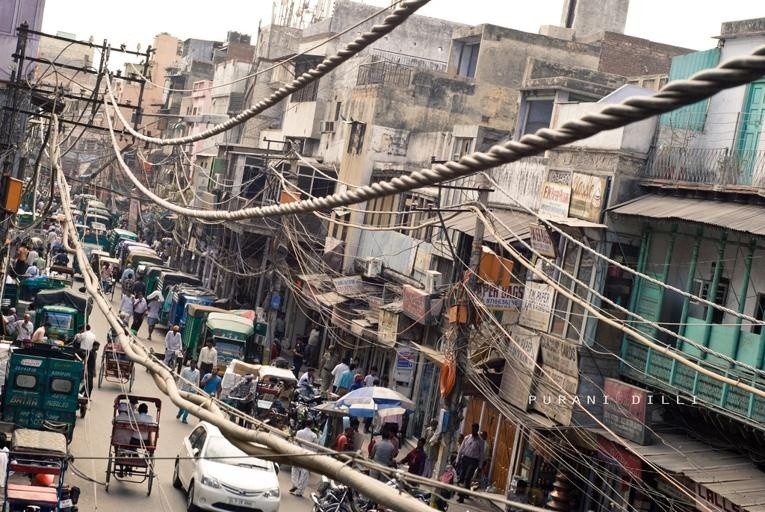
[289,394,330,432]
[309,472,446,512]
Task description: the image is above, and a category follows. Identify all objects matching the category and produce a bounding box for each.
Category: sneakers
[289,486,301,497]
[175,413,188,425]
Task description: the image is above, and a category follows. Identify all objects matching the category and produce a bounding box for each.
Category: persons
[0,240,489,512]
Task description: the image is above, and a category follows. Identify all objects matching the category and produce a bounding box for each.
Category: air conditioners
[211,173,224,191]
[424,270,442,295]
[319,121,337,134]
[363,256,383,278]
